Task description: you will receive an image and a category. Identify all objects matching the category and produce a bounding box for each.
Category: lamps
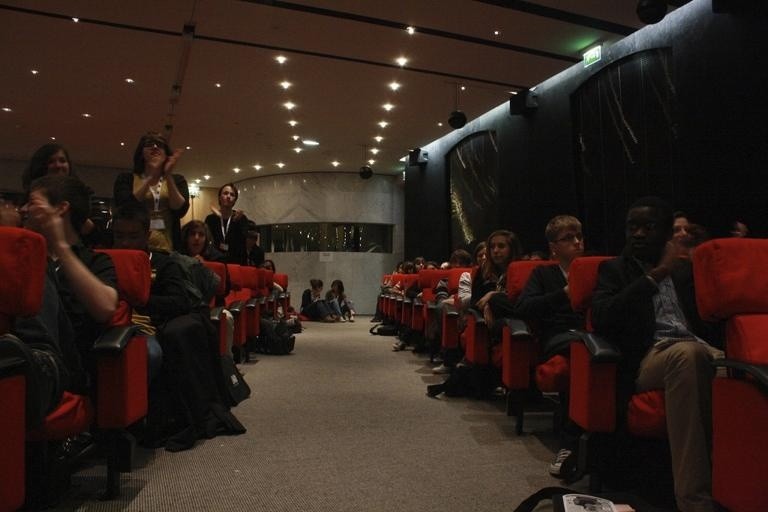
[359,143,375,179]
[447,81,468,129]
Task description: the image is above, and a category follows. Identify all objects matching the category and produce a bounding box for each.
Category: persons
[326,280,357,322]
[300,278,341,322]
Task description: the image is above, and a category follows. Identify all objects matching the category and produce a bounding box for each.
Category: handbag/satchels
[286,317,303,334]
[261,315,295,354]
[370,323,399,335]
[513,486,657,512]
[215,355,255,404]
[427,365,500,397]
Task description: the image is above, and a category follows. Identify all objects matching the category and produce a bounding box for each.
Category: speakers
[510,89,538,115]
[409,147,428,166]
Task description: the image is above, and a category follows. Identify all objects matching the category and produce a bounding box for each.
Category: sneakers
[391,341,405,351]
[550,448,576,476]
[1,333,41,431]
[433,365,449,374]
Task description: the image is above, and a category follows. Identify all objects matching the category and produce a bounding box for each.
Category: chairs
[502,237,767,510]
[368,237,519,403]
[150,249,290,452]
[0,227,151,512]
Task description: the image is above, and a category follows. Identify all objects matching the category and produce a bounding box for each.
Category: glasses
[552,232,585,241]
